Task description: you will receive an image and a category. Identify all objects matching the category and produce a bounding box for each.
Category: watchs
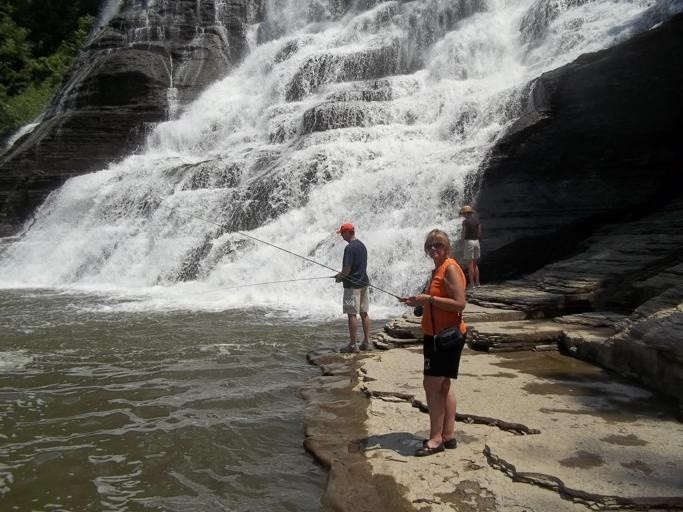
[429,295,433,302]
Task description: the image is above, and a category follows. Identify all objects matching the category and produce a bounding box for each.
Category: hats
[335,224,353,234]
[459,205,474,214]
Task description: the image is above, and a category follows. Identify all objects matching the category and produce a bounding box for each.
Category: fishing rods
[207,276,337,292]
[156,199,407,303]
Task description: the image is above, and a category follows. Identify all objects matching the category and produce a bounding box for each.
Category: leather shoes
[415,439,444,456]
[422,438,456,448]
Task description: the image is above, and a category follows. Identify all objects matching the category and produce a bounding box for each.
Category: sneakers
[358,341,372,351]
[340,342,360,353]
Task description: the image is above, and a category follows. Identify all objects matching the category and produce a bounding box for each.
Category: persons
[398,229,467,456]
[335,224,372,353]
[459,206,481,290]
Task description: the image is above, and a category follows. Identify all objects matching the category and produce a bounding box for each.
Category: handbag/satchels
[432,326,465,353]
